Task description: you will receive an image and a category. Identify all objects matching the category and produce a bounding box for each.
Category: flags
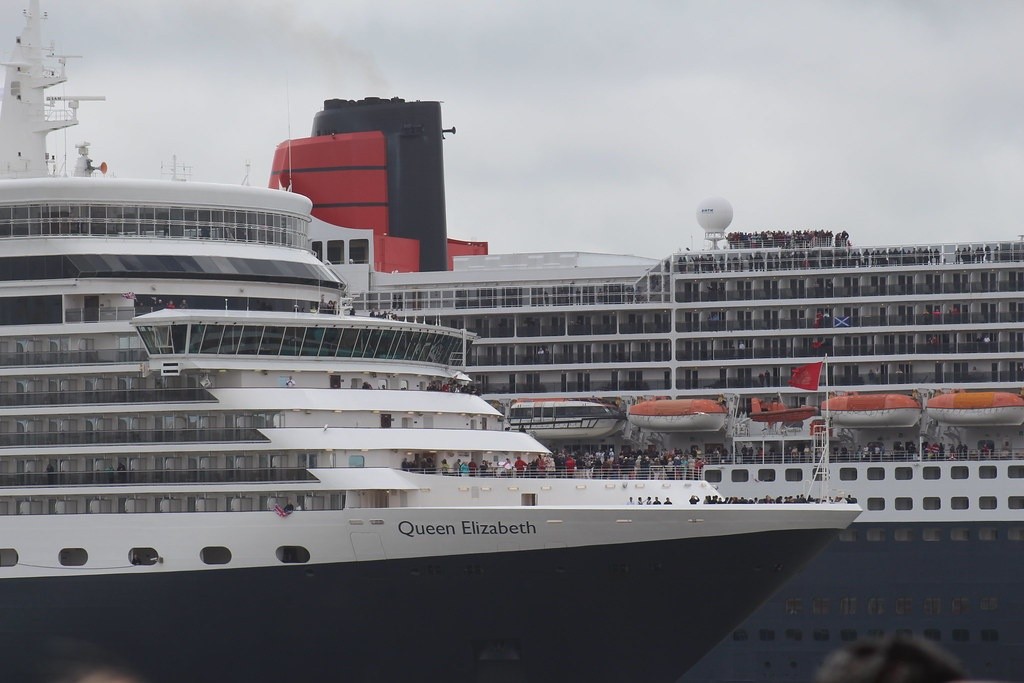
[787,362,824,391]
[121,292,137,300]
[273,505,292,517]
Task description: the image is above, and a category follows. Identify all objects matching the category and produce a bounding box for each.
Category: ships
[0,2,1024,683]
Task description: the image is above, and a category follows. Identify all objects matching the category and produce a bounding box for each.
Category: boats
[504,398,628,441]
[628,397,730,433]
[927,392,1024,426]
[744,396,818,425]
[818,391,922,429]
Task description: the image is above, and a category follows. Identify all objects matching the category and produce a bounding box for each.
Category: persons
[868,369,879,384]
[923,308,959,323]
[816,310,829,320]
[284,500,301,511]
[427,379,481,395]
[759,369,770,387]
[707,313,719,321]
[362,382,372,390]
[105,463,126,483]
[678,229,1000,273]
[286,375,295,387]
[369,309,398,319]
[327,300,334,309]
[46,464,54,484]
[975,336,990,343]
[537,347,544,354]
[350,308,355,315]
[896,369,903,374]
[927,337,936,344]
[739,341,745,350]
[401,441,992,505]
[158,299,188,310]
